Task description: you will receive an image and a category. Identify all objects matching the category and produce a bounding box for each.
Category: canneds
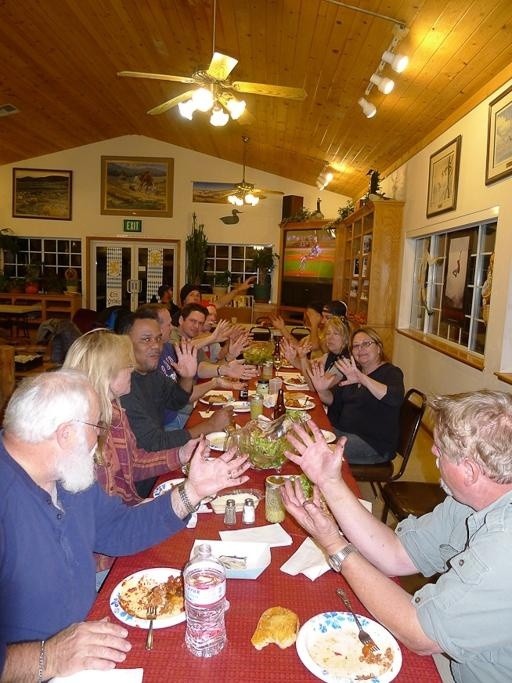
[257,380,269,395]
[251,393,263,418]
[263,361,273,380]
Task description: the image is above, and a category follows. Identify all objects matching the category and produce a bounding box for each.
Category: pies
[251,607,300,650]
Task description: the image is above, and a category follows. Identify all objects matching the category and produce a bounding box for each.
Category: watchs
[329,543,355,572]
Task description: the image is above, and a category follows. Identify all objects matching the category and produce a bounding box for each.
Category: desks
[0,305,40,338]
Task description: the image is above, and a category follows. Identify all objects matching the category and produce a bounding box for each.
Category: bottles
[180,543,228,657]
[223,499,237,526]
[239,362,248,399]
[243,498,255,524]
[275,389,288,417]
[273,340,281,360]
[256,379,269,394]
[249,393,264,418]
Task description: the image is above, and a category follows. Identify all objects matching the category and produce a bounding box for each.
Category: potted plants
[185,213,280,303]
[0,260,80,294]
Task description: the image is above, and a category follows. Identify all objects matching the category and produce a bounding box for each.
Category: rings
[229,472,232,479]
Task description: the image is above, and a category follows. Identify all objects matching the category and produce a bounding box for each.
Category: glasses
[352,340,376,351]
[75,419,111,440]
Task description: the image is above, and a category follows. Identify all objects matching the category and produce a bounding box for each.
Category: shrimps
[365,169,377,193]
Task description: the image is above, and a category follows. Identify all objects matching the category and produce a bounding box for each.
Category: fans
[213,136,285,200]
[116,0,307,115]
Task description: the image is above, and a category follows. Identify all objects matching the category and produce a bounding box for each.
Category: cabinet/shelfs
[0,292,81,323]
[341,200,404,363]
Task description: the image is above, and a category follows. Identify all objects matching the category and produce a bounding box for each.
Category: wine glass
[273,357,283,376]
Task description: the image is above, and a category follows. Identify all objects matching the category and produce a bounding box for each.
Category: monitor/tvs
[279,220,339,284]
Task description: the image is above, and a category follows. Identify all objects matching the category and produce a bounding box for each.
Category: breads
[204,395,228,402]
[285,398,301,407]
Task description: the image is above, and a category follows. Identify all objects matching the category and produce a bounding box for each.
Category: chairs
[249,326,271,343]
[382,479,447,524]
[349,387,427,498]
[291,327,310,340]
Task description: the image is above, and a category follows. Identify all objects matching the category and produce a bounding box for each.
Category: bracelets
[179,484,201,513]
[39,640,44,683]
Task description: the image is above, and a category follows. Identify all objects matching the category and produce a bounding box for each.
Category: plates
[199,394,251,412]
[285,398,316,410]
[109,566,188,629]
[204,430,241,451]
[153,477,216,504]
[321,429,336,443]
[285,378,308,387]
[295,610,405,682]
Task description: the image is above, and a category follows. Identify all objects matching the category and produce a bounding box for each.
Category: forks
[335,586,379,651]
[144,605,157,651]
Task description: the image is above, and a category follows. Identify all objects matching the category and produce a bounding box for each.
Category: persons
[306,327,405,465]
[113,309,233,498]
[277,390,512,683]
[60,328,211,592]
[1,369,253,683]
[268,300,354,393]
[139,277,256,430]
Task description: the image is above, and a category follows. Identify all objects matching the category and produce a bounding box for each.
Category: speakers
[281,195,303,222]
[283,281,332,307]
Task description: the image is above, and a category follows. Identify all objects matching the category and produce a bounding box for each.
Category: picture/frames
[12,167,72,221]
[485,84,512,186]
[100,155,174,218]
[426,134,463,219]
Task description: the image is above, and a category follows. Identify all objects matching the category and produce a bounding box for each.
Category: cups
[231,313,238,324]
[262,366,272,381]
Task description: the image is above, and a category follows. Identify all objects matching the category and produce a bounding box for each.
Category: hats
[181,283,194,299]
[158,284,170,296]
[322,299,348,320]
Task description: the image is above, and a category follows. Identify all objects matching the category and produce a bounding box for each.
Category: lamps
[357,24,411,119]
[177,85,246,127]
[316,163,333,191]
[227,193,259,206]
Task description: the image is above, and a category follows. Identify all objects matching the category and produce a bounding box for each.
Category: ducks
[219,209,243,224]
